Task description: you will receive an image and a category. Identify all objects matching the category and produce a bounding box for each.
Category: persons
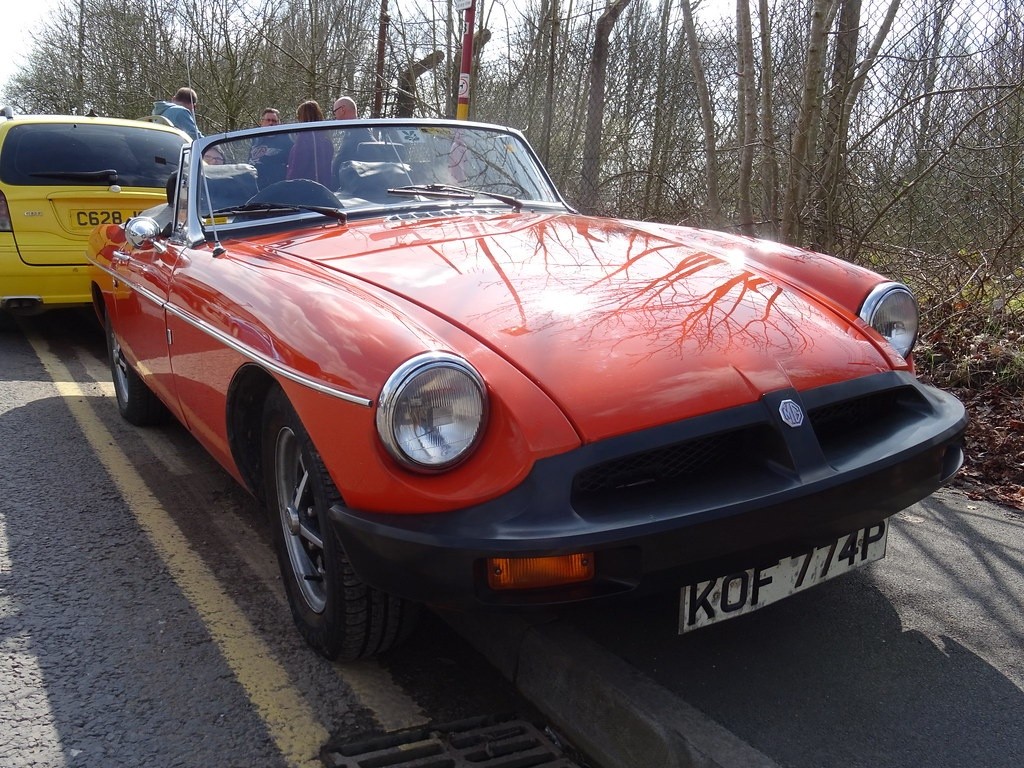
[247,108,295,192]
[137,168,188,238]
[204,145,226,165]
[152,87,205,140]
[333,97,378,192]
[286,100,334,192]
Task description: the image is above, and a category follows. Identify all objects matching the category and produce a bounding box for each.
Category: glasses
[262,118,278,124]
[204,155,224,162]
[332,105,345,116]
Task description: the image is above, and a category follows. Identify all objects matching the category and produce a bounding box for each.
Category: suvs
[0,105,229,318]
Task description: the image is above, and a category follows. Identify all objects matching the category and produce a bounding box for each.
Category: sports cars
[84,118,970,665]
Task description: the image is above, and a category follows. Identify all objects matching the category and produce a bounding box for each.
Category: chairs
[167,163,259,214]
[335,161,414,206]
